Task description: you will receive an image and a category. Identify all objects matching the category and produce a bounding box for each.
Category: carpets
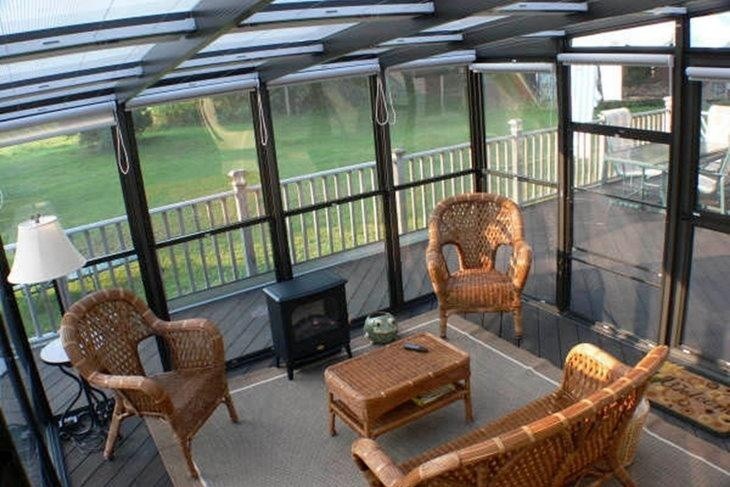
[643,359,730,437]
[142,308,730,487]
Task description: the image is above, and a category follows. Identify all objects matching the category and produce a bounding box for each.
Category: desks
[39,331,116,454]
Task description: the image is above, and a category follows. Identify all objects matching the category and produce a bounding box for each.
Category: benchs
[350,343,669,487]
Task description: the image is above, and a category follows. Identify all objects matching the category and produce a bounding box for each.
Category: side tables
[262,268,353,381]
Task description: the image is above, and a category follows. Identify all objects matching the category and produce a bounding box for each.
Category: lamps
[7,212,88,317]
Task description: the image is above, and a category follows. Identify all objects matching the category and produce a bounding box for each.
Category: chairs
[598,96,730,216]
[59,288,239,478]
[425,192,533,339]
